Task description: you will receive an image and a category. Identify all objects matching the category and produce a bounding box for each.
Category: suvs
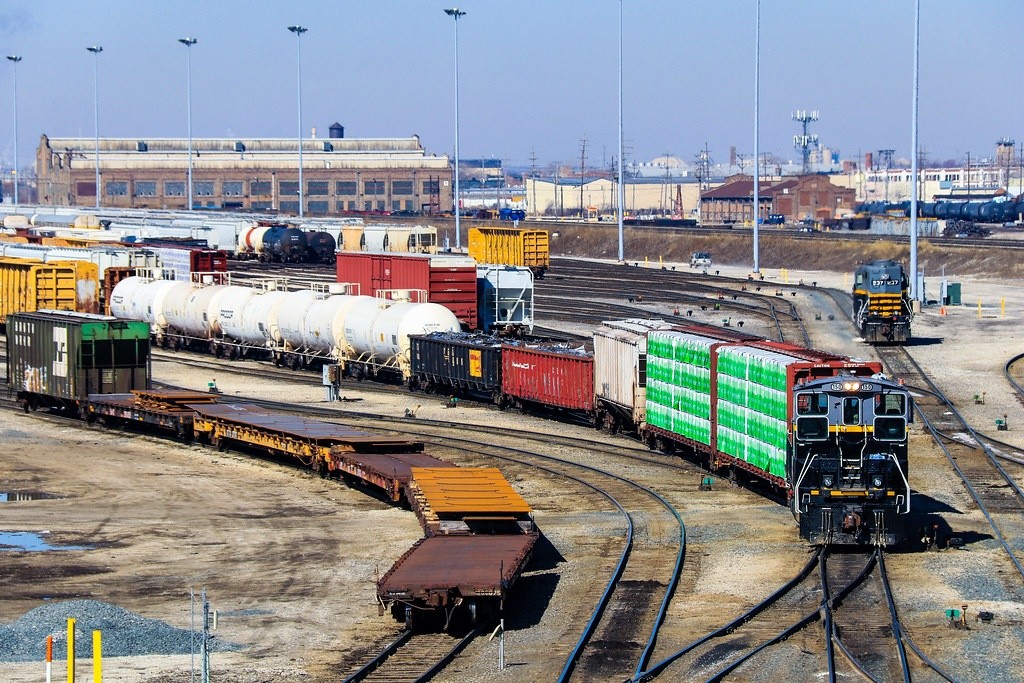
[765,213,785,224]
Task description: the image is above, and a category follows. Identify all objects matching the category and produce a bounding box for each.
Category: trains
[856,201,1004,224]
[0,305,542,628]
[998,201,1024,222]
[851,257,915,345]
[0,201,920,555]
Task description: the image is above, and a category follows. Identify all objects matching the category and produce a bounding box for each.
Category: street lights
[85,45,104,209]
[177,36,198,211]
[442,8,467,251]
[5,55,23,213]
[965,151,971,203]
[287,24,309,219]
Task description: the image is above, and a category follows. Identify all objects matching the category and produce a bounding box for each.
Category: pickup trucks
[689,252,712,268]
